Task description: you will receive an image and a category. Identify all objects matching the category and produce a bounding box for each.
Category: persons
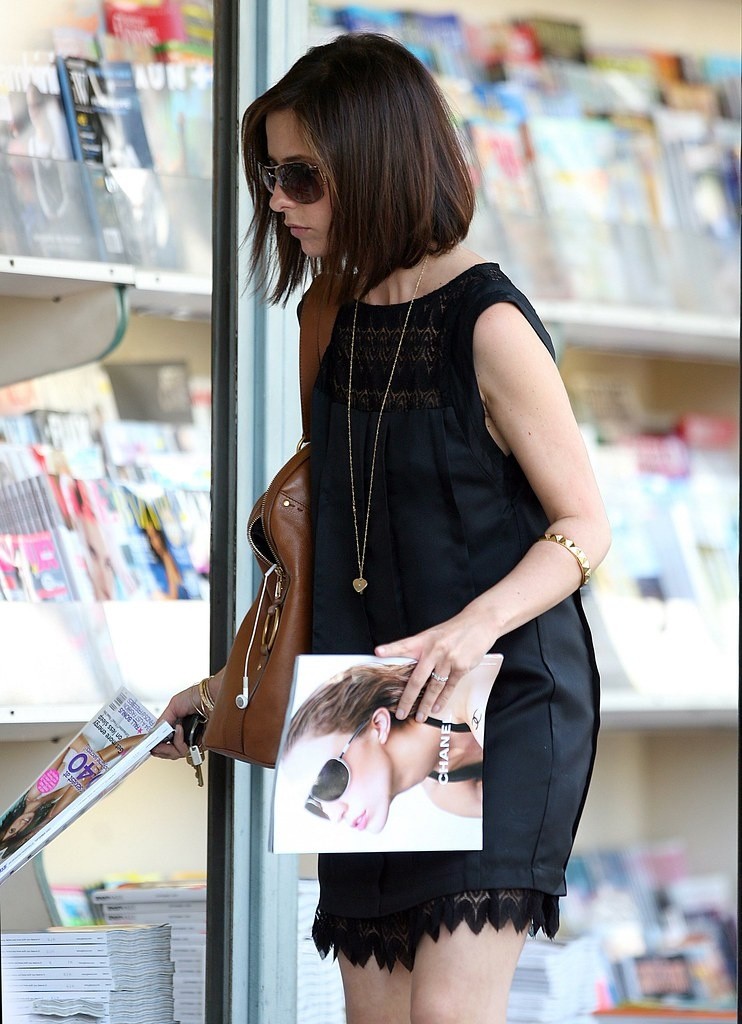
[151,32,611,1024]
[0,735,102,851]
[275,661,488,837]
[2,734,145,859]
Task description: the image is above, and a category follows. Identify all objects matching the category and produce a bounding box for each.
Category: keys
[186,748,205,787]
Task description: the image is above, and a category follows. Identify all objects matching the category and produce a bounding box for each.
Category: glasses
[256,160,328,204]
[304,718,372,820]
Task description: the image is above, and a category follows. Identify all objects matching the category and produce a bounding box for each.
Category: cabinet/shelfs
[0,3,741,1023]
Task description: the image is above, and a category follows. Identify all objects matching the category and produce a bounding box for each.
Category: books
[325,6,737,1024]
[0,0,221,1024]
[267,654,504,852]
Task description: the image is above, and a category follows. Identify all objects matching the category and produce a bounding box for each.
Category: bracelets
[540,534,592,587]
[190,676,216,720]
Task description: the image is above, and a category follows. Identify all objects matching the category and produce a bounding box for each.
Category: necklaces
[347,253,430,596]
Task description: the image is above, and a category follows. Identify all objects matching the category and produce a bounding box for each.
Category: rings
[431,672,449,682]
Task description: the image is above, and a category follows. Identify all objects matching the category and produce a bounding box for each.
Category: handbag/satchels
[201,274,343,770]
